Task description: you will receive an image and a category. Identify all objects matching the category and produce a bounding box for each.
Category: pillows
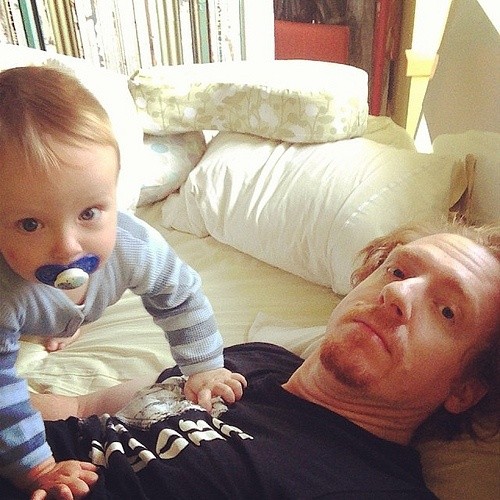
[0,44,145,215]
[158,128,476,295]
[125,58,368,144]
[137,129,206,205]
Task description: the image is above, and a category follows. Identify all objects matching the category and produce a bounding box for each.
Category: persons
[0,64,249,500]
[0,214,500,499]
